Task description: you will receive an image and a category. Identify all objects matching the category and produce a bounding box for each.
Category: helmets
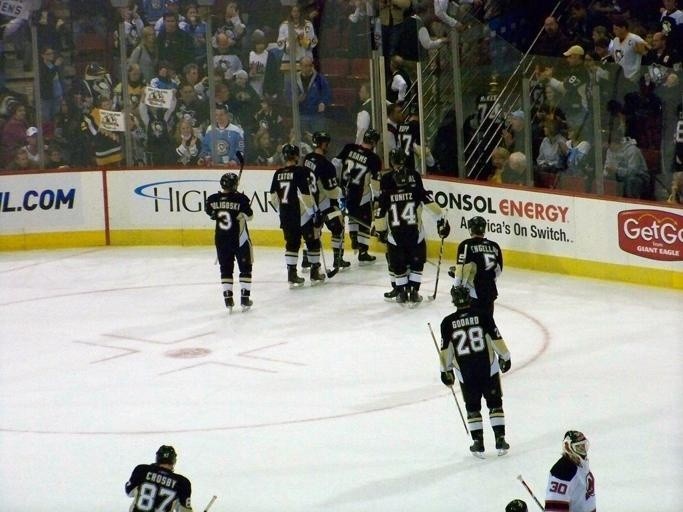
[450,285,472,308]
[219,173,239,192]
[312,130,331,144]
[467,215,487,236]
[562,430,591,468]
[388,147,406,167]
[282,142,301,155]
[362,128,380,146]
[154,445,178,465]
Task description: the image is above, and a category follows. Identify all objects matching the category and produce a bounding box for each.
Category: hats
[561,45,586,57]
[510,109,525,120]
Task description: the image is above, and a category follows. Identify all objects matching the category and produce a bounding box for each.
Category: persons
[269,126,450,310]
[544,431,595,512]
[125,445,198,512]
[506,500,529,512]
[448,214,504,313]
[1,0,683,205]
[440,288,512,458]
[204,174,254,313]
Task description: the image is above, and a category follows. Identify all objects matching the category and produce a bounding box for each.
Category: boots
[409,286,424,303]
[223,289,234,307]
[332,253,351,267]
[310,265,326,280]
[241,294,253,306]
[494,428,510,450]
[302,255,312,267]
[468,438,486,453]
[287,269,305,284]
[358,249,377,262]
[396,286,409,301]
[383,286,399,298]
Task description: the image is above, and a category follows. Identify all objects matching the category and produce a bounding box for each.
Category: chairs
[0,1,683,206]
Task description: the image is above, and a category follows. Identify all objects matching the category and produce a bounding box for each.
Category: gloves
[497,355,511,374]
[440,370,456,386]
[375,228,389,242]
[434,216,451,240]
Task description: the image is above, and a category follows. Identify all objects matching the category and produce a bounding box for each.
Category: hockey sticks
[428,208,448,300]
[214,150,245,264]
[327,198,346,278]
[319,236,331,274]
